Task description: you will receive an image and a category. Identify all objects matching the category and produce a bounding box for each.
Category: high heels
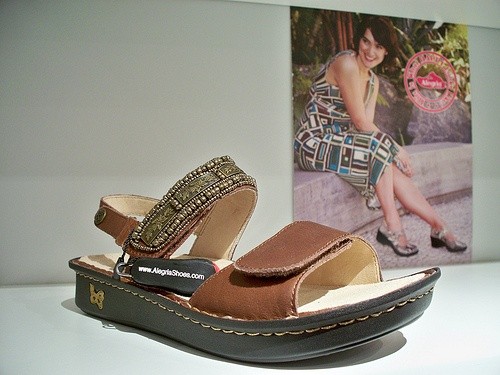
[429,224,467,254]
[376,219,419,257]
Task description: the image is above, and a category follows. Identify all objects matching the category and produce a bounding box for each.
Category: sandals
[67,155,442,365]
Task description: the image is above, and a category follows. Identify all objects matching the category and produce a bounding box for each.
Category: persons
[294,16,468,257]
